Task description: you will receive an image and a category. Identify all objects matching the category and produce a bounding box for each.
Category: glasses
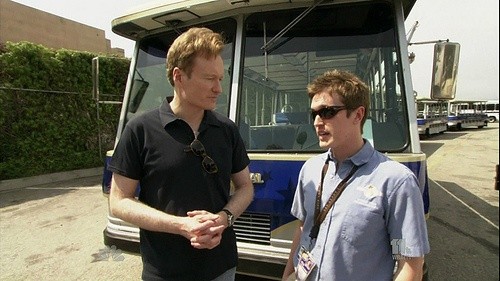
[312,104,357,121]
[190,139,219,176]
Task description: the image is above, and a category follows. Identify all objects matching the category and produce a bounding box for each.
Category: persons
[107,26,255,281]
[281,74,431,281]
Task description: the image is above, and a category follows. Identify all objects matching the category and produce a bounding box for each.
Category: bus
[91,0,460,281]
[414,100,499,139]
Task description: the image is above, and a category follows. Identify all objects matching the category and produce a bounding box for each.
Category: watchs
[223,209,235,226]
